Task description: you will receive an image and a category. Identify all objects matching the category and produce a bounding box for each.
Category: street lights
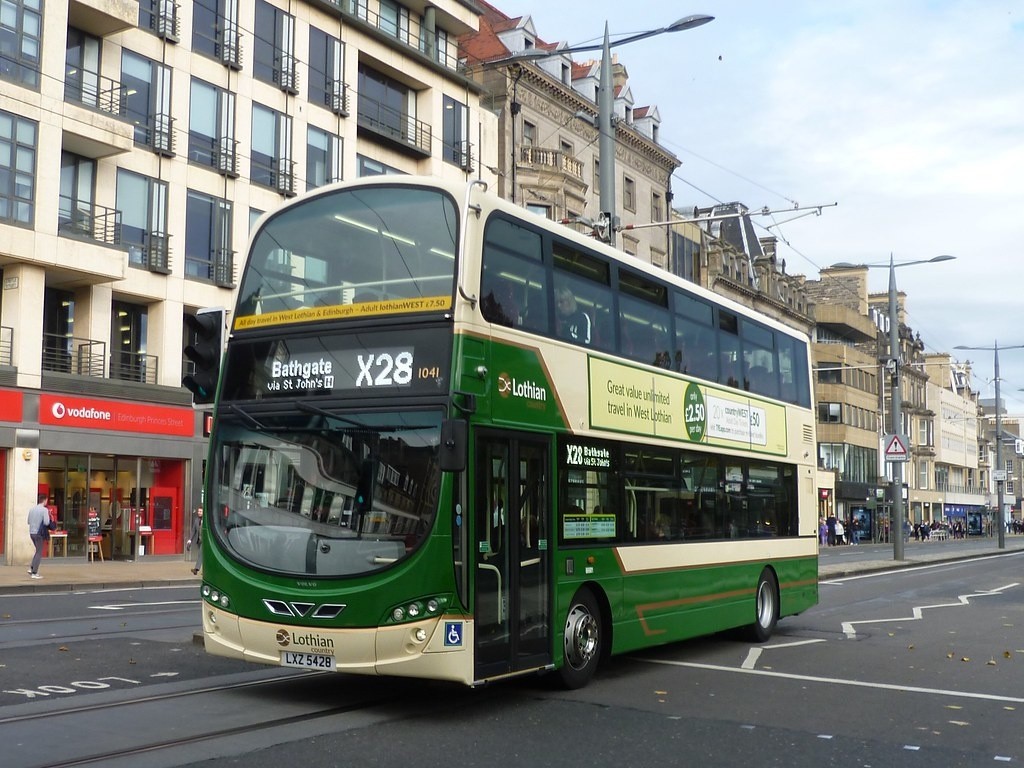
[478,15,715,246]
[951,339,1024,549]
[831,252,957,561]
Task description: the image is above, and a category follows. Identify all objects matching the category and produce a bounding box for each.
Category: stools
[88,540,105,564]
[126,531,154,555]
[47,534,68,557]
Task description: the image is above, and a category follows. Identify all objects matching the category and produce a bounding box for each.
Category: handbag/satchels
[187,544,192,551]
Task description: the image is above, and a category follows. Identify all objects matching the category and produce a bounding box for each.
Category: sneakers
[28,568,44,579]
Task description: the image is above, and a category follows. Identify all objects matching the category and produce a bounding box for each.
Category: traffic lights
[182,307,226,410]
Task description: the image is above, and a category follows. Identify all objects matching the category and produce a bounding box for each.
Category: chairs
[522,304,796,403]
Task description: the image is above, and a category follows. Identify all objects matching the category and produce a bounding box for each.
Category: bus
[201,175,819,690]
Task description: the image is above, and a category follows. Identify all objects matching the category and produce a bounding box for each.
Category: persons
[1007,517,1024,535]
[186,506,203,575]
[818,514,863,546]
[26,493,53,581]
[910,518,968,544]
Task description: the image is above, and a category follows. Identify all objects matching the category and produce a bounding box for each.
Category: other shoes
[191,569,198,575]
